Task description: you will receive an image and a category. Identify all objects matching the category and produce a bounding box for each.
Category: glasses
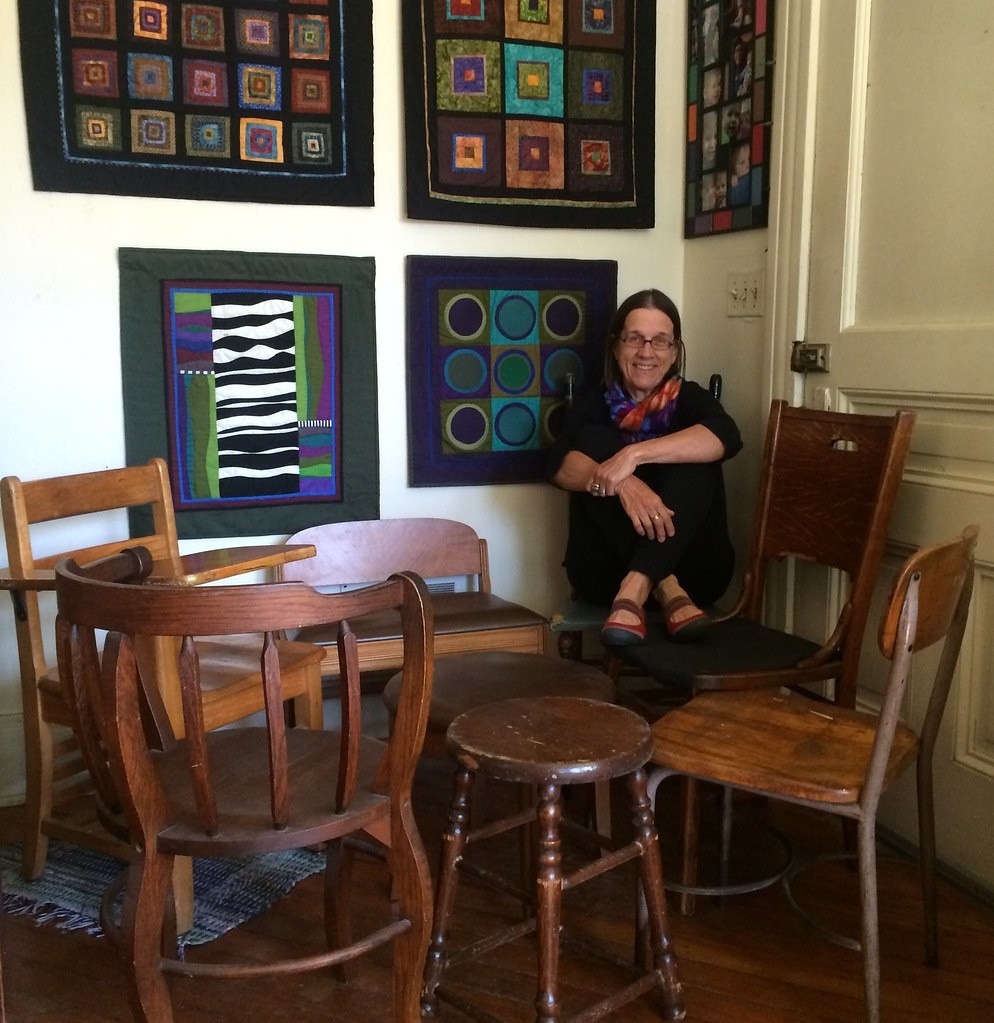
[619,333,674,351]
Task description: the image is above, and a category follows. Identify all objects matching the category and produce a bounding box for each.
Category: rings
[651,514,660,521]
[591,483,599,492]
[599,489,605,494]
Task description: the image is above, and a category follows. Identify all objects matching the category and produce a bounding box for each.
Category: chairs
[549,374,727,800]
[54,546,433,1022]
[276,515,548,729]
[630,524,980,1023]
[608,399,916,913]
[0,459,327,937]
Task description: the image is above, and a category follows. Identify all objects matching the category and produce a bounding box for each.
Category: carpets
[0,808,328,960]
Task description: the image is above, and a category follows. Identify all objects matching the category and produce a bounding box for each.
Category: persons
[542,289,743,643]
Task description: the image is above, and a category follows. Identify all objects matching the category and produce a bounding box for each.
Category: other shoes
[664,595,712,646]
[601,598,646,644]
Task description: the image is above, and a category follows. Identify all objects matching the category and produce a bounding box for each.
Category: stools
[419,697,687,1023]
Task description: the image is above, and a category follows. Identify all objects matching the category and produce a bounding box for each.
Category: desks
[383,650,621,935]
